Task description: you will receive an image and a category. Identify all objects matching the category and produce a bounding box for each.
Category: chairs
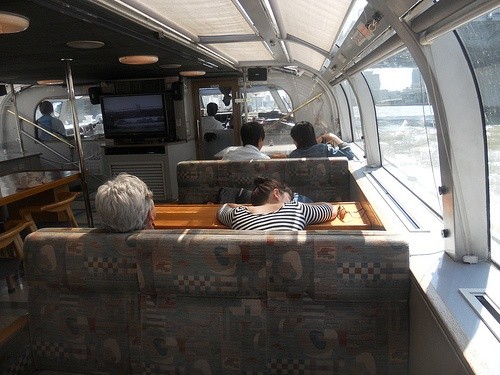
[0,192,80,293]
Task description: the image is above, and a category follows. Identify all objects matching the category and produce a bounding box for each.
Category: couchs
[177,157,350,204]
[21,228,410,375]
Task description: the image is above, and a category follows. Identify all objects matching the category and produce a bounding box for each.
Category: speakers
[172,82,184,100]
[88,87,102,105]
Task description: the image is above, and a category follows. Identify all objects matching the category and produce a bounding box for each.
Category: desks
[153,201,372,231]
[0,170,81,207]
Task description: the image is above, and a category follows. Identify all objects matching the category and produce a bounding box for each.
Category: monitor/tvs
[100,93,176,145]
